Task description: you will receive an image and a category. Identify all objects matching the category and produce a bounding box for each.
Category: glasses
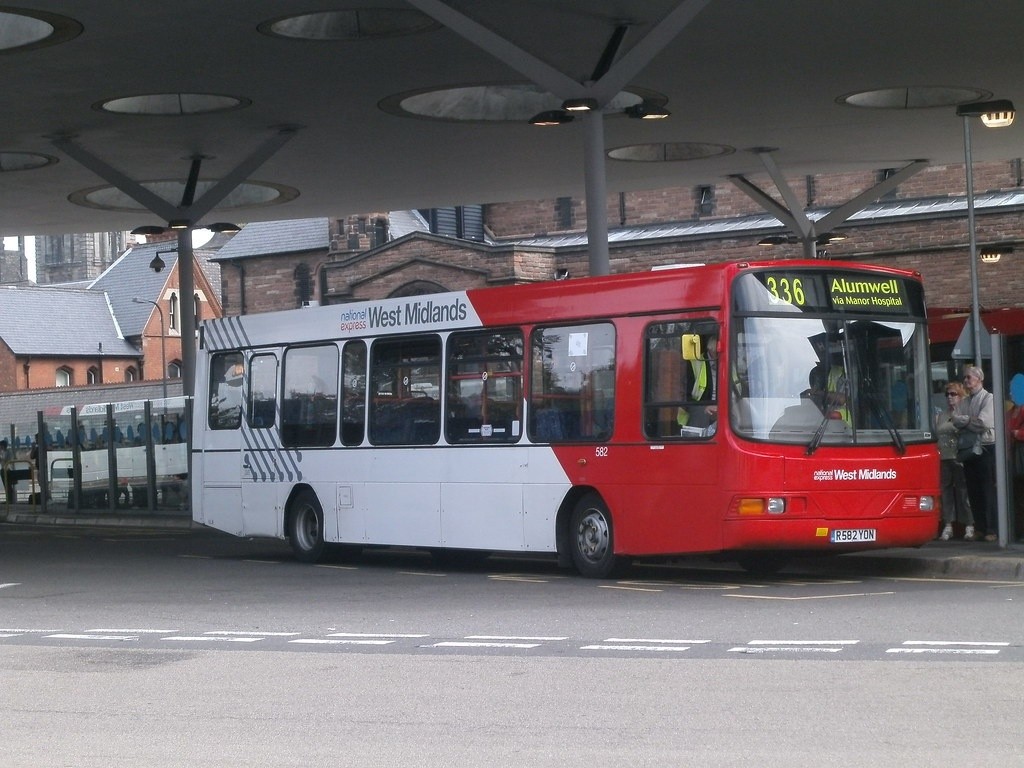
[963,375,977,380]
[945,391,958,397]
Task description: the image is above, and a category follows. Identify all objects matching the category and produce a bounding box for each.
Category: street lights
[133,294,170,518]
[954,100,1017,551]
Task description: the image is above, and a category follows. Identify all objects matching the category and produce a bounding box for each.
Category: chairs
[404,387,585,444]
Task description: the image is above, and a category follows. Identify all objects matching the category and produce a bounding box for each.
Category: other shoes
[4,500,12,504]
[966,531,984,541]
[983,532,998,541]
[964,525,977,540]
[940,527,953,541]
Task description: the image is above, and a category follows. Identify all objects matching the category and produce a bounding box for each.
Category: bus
[191,259,941,582]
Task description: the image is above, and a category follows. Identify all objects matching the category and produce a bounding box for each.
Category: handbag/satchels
[956,431,983,463]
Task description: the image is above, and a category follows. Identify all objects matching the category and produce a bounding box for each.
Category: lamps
[130,225,165,234]
[626,102,671,119]
[817,234,850,242]
[979,246,1015,264]
[167,222,193,230]
[561,97,597,111]
[758,237,803,245]
[208,222,242,234]
[527,112,573,126]
[149,249,178,272]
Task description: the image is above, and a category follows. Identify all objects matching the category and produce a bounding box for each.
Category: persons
[30,434,49,504]
[935,383,974,541]
[808,365,853,429]
[0,440,13,504]
[1006,400,1024,540]
[677,335,717,428]
[952,367,998,542]
